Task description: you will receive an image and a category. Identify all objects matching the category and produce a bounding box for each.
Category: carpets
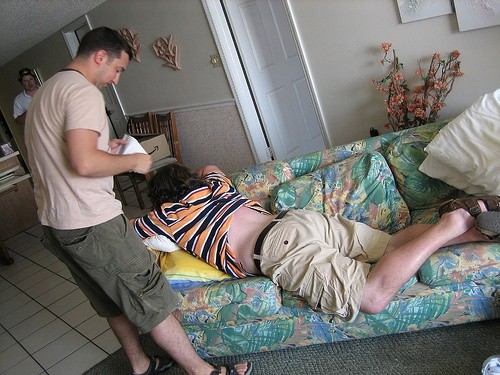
[82,318,500,375]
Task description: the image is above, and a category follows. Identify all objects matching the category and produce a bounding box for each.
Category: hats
[18,68,35,82]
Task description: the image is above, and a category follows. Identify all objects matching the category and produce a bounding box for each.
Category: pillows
[424,87,500,197]
[144,234,236,289]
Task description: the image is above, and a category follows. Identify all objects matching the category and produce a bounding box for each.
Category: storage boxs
[131,133,170,163]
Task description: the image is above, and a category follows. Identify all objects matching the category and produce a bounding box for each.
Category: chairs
[113,112,184,209]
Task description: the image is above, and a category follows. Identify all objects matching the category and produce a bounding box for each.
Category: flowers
[371,42,464,132]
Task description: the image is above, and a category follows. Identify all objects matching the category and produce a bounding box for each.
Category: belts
[254,210,290,277]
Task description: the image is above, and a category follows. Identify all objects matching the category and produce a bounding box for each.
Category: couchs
[169,118,500,358]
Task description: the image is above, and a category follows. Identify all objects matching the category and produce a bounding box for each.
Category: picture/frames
[31,69,42,87]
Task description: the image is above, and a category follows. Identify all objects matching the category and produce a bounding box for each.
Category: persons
[130,164,500,324]
[24,25,253,375]
[13,68,42,125]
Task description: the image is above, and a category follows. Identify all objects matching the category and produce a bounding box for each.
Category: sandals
[438,196,500,241]
[131,355,174,375]
[210,361,253,375]
